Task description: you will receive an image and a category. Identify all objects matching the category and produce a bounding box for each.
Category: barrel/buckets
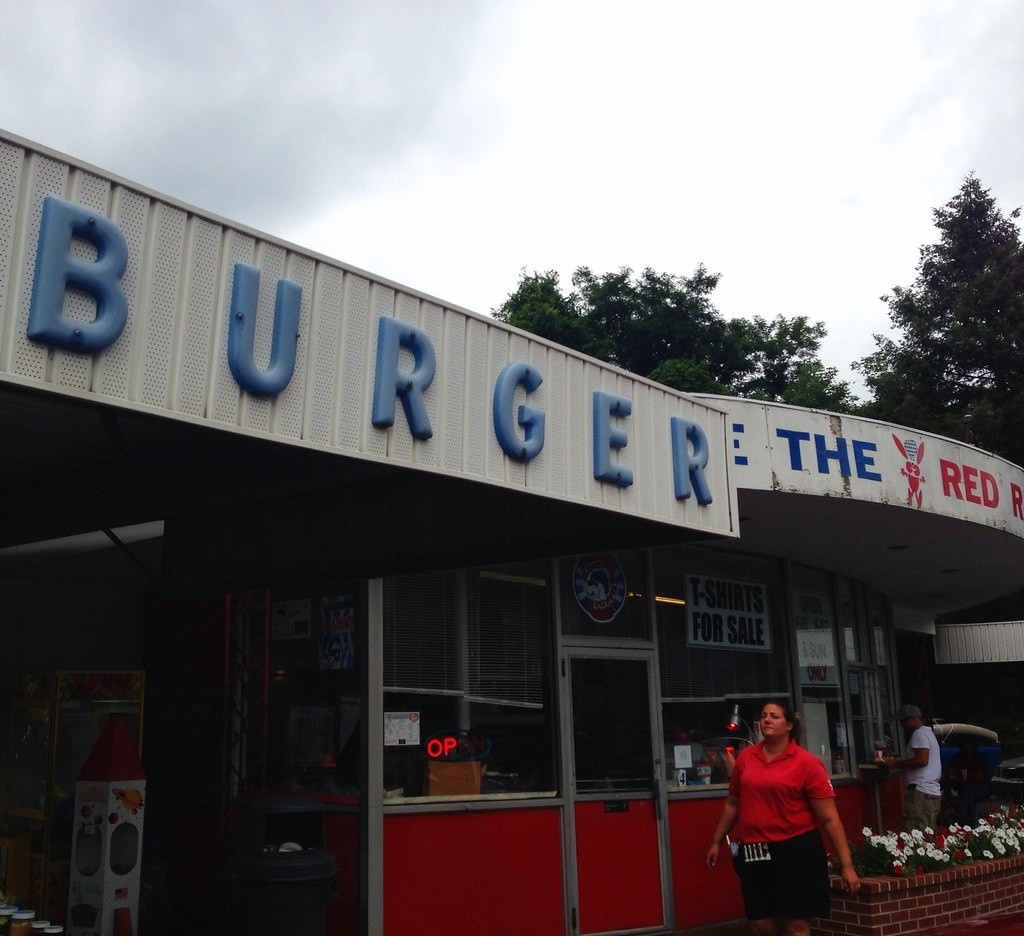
[235,844,343,936]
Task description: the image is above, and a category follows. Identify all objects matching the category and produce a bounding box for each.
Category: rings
[859,883,861,887]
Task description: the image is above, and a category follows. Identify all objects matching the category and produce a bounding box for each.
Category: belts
[906,784,917,790]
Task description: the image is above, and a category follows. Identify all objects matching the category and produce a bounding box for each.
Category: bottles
[833,754,845,775]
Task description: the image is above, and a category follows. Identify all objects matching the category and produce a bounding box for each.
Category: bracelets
[712,836,722,842]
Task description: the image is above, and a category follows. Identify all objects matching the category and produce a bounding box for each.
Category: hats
[891,704,921,720]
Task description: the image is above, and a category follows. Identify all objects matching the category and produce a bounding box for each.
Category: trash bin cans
[227,795,340,936]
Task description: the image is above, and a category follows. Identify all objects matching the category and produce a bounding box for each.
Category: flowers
[825,801,1024,876]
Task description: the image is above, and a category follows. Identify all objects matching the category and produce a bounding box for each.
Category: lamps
[725,704,757,744]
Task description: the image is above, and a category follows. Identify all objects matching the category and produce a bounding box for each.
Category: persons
[881,703,942,829]
[948,740,992,793]
[705,700,861,936]
